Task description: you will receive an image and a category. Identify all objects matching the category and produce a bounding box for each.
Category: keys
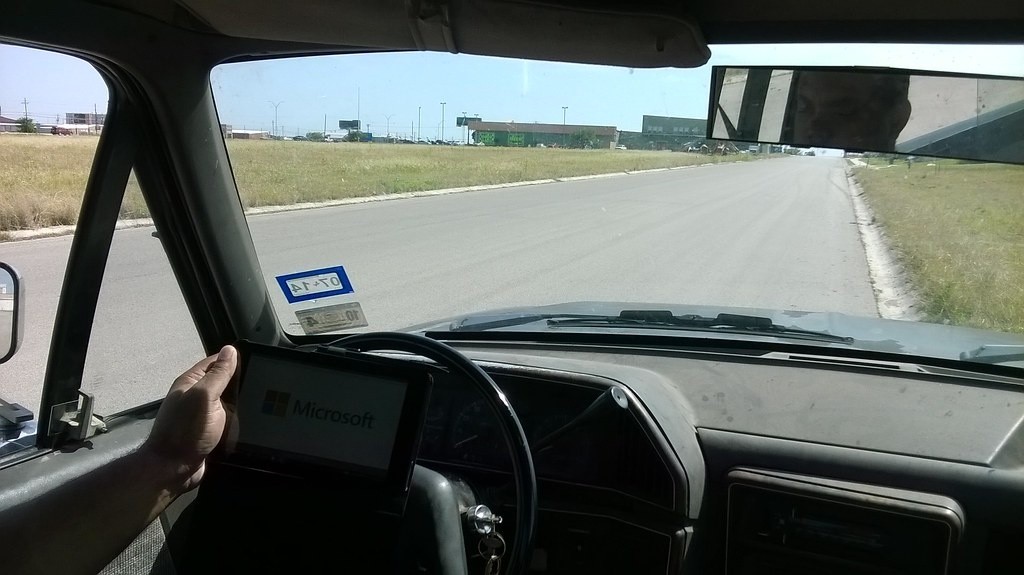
[482,533,503,556]
[478,514,500,525]
[484,557,499,575]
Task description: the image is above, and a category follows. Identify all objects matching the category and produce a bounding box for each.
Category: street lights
[440,103,446,141]
[419,107,422,138]
[562,106,568,125]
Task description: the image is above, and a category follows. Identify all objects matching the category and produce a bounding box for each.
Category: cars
[615,144,627,150]
[397,137,465,146]
[270,134,309,142]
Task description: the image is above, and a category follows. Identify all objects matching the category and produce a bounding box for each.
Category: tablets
[208,339,434,521]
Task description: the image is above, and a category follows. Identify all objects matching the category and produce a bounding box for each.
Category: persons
[794,70,911,150]
[0,343,238,575]
